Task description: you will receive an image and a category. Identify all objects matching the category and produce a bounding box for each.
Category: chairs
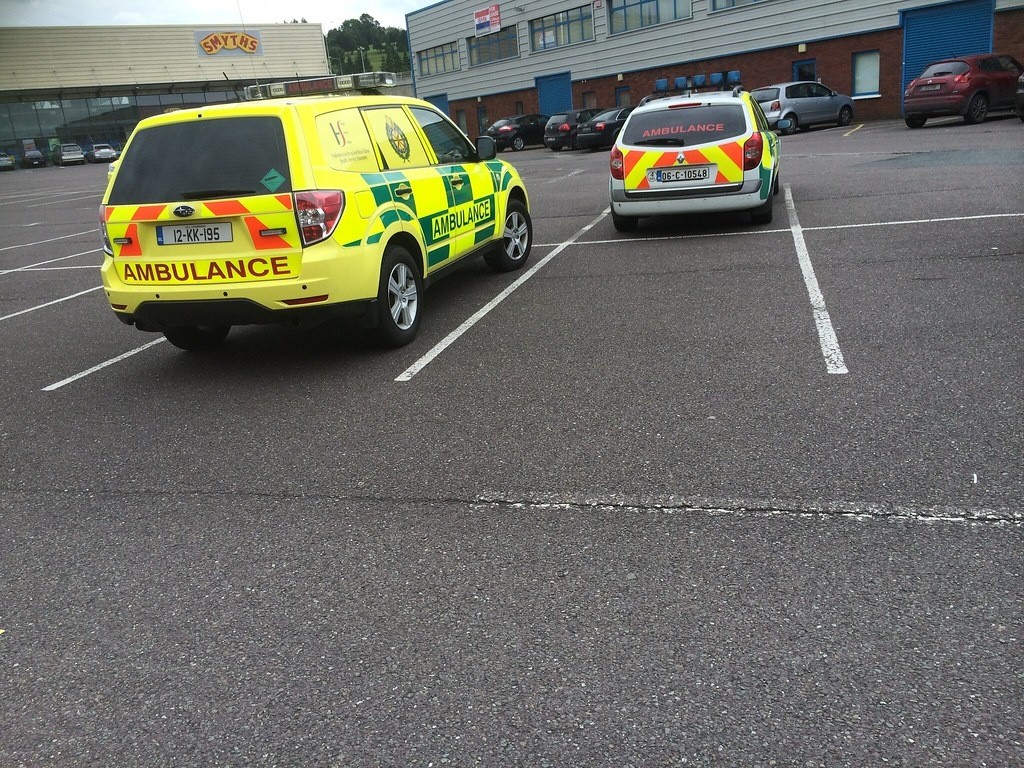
[674,76,688,91]
[652,79,669,94]
[710,72,725,87]
[727,71,742,85]
[693,74,707,89]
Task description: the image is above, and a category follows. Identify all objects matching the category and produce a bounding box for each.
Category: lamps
[12,71,24,102]
[92,68,102,98]
[264,63,274,83]
[165,66,175,94]
[53,70,64,99]
[232,64,243,91]
[198,64,209,93]
[129,67,140,96]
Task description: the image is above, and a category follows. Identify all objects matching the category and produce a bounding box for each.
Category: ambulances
[95,71,534,353]
[607,84,793,234]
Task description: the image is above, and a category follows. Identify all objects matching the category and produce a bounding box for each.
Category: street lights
[329,57,343,75]
[357,46,367,73]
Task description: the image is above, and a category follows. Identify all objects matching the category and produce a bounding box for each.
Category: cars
[750,80,858,135]
[486,114,551,154]
[544,107,606,152]
[85,143,118,163]
[575,104,638,152]
[0,152,14,170]
[19,150,44,168]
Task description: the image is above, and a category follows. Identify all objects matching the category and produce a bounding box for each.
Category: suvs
[903,52,1024,129]
[52,143,86,166]
